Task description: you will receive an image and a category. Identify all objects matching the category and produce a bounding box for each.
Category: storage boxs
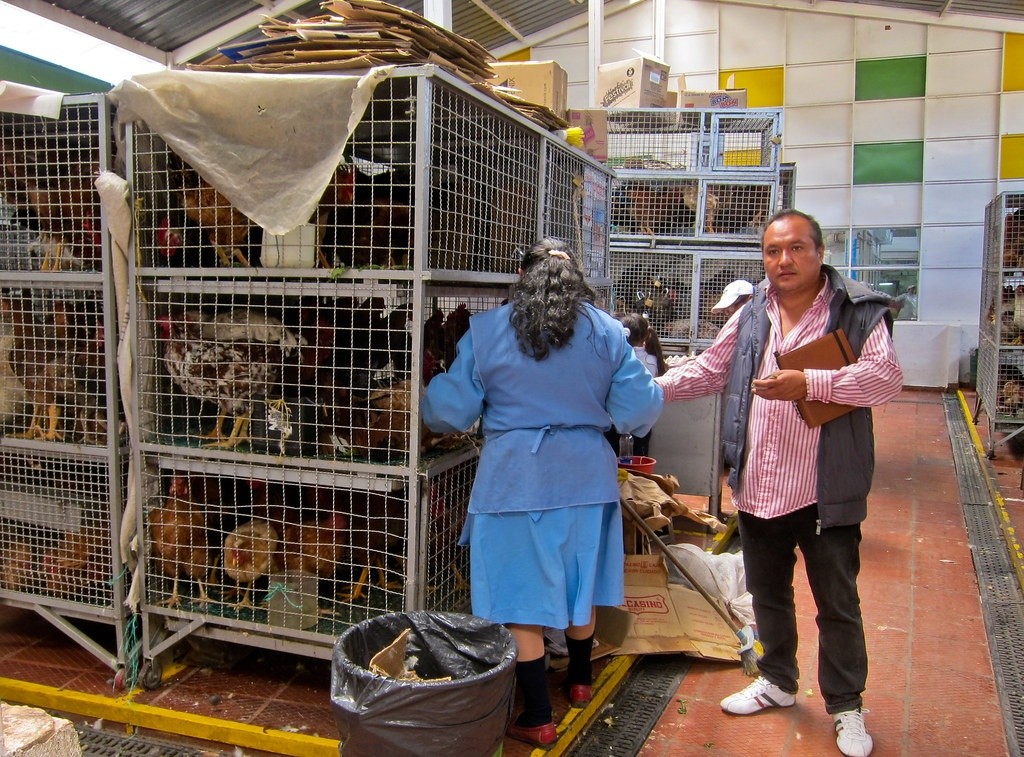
[488,56,748,162]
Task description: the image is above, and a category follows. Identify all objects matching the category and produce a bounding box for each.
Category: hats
[906,285,915,290]
[712,280,754,313]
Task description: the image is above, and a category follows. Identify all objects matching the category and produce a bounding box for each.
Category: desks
[893,320,964,392]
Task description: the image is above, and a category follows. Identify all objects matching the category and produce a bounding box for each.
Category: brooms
[620,496,764,677]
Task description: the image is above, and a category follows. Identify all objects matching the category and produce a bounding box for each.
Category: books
[777,329,860,428]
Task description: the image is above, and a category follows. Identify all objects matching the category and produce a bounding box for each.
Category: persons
[608,313,665,460]
[420,238,664,750]
[711,280,755,319]
[652,209,904,757]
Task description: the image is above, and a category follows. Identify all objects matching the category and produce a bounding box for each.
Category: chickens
[141,470,468,607]
[612,261,736,340]
[0,289,476,460]
[987,203,1024,414]
[609,159,770,238]
[1,479,117,598]
[0,128,526,272]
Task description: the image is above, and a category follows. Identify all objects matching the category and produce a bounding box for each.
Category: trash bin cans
[334,610,519,757]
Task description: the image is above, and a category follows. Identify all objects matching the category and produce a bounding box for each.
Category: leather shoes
[505,710,557,746]
[567,684,592,709]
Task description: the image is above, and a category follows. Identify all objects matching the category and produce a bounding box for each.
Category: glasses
[724,298,745,315]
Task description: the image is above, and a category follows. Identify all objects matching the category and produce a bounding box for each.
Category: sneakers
[720,675,796,714]
[832,705,873,757]
